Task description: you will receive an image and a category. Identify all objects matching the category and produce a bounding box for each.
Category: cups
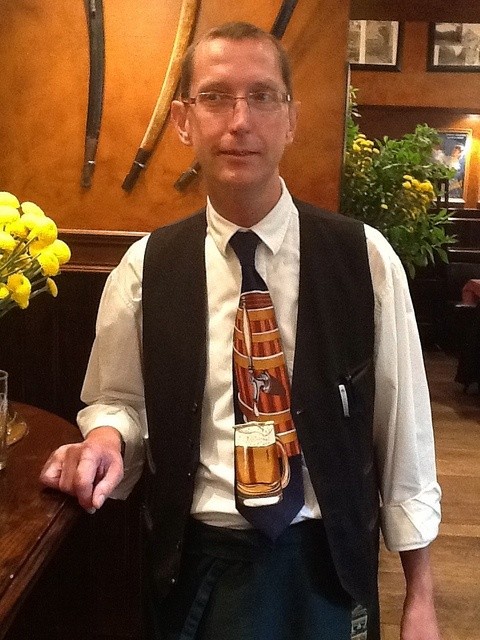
[232,421,290,507]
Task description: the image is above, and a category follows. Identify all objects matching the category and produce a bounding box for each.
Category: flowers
[343,133,380,178]
[1,188,73,320]
[373,175,436,226]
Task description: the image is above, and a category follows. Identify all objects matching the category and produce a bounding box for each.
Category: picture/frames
[427,127,474,205]
[347,16,405,73]
[425,18,480,73]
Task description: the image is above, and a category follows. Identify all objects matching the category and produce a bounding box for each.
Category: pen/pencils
[333,381,352,424]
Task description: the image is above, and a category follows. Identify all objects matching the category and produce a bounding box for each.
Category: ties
[228,230,305,544]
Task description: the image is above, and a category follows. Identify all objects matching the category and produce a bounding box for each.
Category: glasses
[178,91,293,114]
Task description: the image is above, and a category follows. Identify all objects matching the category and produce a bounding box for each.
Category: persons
[38,22,443,640]
[442,144,462,171]
